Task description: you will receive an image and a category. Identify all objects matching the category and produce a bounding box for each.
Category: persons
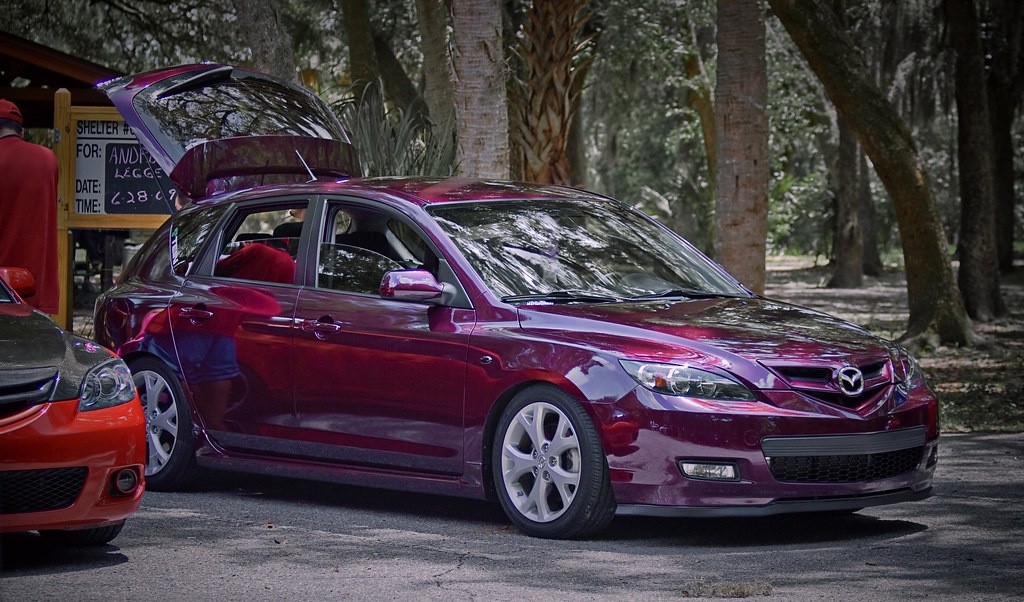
[0,100,59,314]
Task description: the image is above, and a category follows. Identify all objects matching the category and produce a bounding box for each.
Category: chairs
[231,220,408,294]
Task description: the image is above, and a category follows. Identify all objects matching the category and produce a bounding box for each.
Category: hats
[0,100,23,125]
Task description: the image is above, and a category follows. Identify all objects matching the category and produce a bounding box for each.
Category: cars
[91,63,941,541]
[1,266,147,555]
